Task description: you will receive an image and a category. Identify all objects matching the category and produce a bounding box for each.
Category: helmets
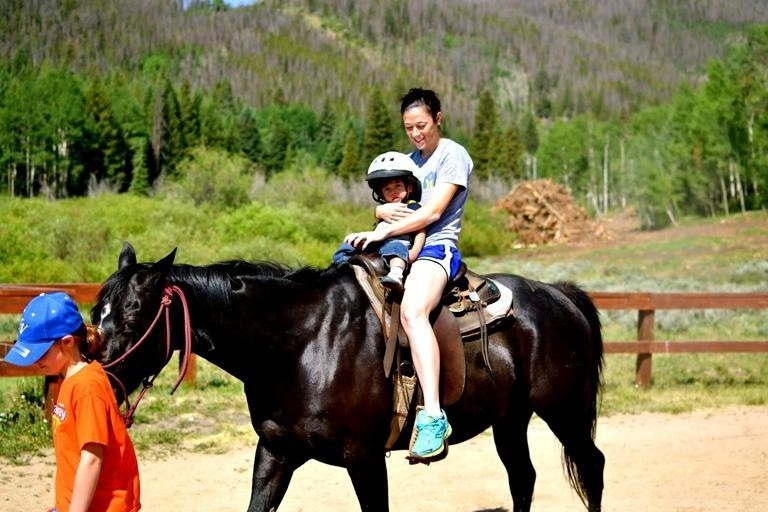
[365,150,423,203]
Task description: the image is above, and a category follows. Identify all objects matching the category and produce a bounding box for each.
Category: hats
[2,292,86,367]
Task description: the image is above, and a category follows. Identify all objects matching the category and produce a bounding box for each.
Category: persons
[344,88,474,459]
[335,150,426,292]
[3,291,141,512]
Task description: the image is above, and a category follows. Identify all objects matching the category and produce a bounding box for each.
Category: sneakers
[379,270,405,292]
[409,407,453,460]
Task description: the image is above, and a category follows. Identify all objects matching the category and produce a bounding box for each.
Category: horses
[88,240,605,512]
